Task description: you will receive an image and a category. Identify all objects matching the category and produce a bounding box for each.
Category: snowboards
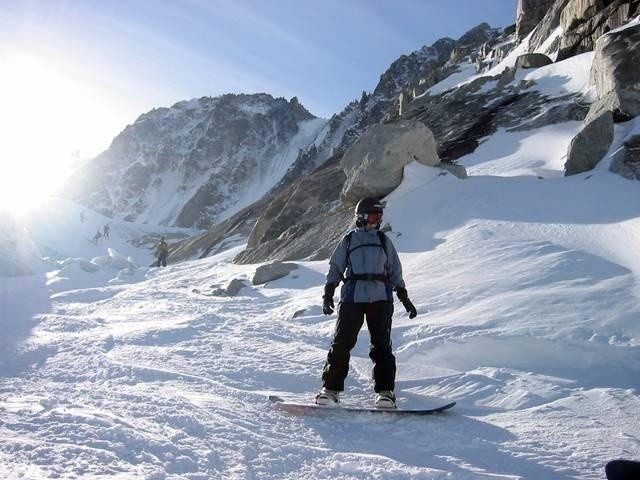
[269,395,456,415]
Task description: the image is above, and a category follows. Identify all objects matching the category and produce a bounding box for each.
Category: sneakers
[375,390,396,408]
[315,387,340,406]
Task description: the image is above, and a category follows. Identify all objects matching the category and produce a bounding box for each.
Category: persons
[103,223,112,238]
[153,236,167,267]
[91,229,103,246]
[311,195,419,407]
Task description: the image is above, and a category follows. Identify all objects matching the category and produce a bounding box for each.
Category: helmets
[355,196,383,227]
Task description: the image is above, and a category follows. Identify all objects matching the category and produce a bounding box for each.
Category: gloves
[322,283,334,315]
[393,286,417,319]
